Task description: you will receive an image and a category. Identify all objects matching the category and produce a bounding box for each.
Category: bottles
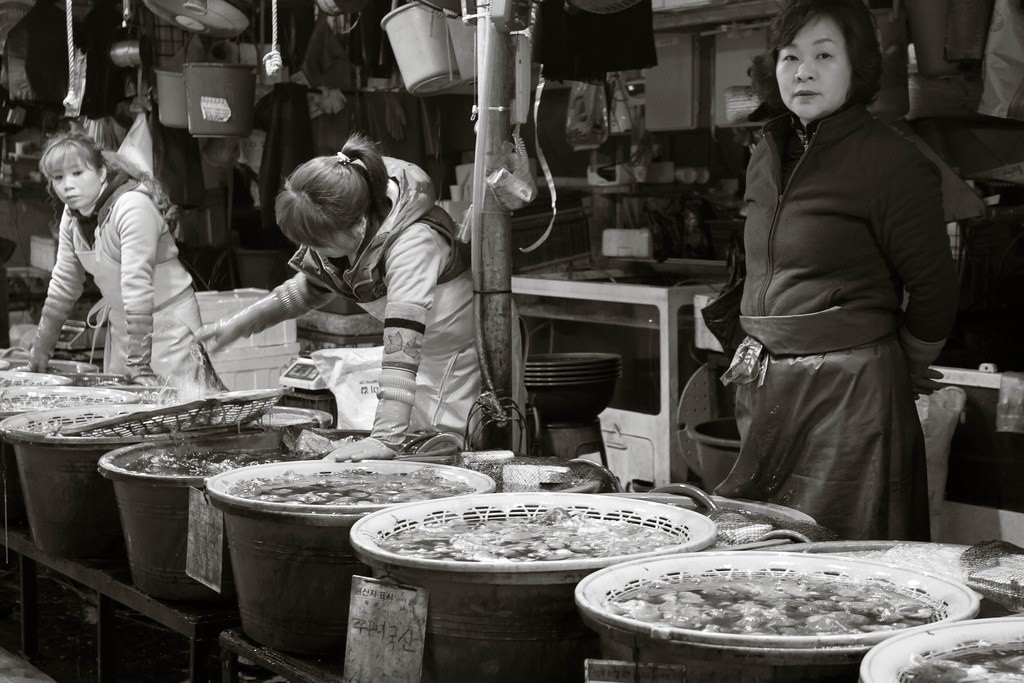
[564,81,608,150]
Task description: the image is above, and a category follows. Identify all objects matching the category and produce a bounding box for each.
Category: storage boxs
[30,234,59,273]
[190,288,300,393]
[602,228,653,257]
[181,206,225,249]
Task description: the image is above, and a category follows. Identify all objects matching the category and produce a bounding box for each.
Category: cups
[484,153,538,212]
[674,167,710,184]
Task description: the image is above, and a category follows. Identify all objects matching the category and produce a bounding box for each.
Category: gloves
[27,301,72,372]
[896,324,948,399]
[193,281,310,353]
[332,368,417,462]
[122,313,170,388]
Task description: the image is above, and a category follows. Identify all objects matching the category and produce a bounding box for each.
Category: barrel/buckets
[185,62,257,140]
[153,64,188,129]
[380,2,461,98]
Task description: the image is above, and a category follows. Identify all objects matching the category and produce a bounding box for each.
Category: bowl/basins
[522,350,622,428]
[686,417,741,496]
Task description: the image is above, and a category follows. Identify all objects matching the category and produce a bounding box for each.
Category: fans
[143,0,250,38]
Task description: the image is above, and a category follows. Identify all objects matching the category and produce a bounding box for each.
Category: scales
[55,319,108,352]
[279,358,333,395]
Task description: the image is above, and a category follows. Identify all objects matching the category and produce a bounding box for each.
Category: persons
[191,132,484,463]
[713,1,962,541]
[14,131,229,392]
